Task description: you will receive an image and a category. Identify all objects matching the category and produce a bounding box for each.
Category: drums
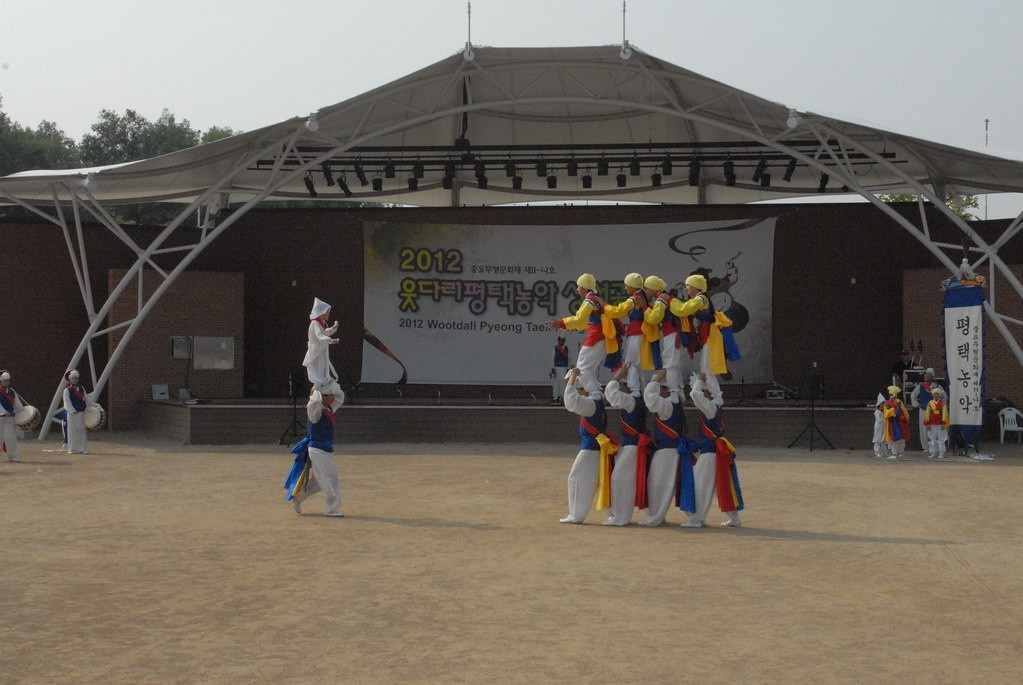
[15,405,41,431]
[83,406,106,430]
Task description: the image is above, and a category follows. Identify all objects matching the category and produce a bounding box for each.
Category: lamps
[304,162,855,198]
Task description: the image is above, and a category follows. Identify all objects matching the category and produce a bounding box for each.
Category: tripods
[788,401,836,453]
[278,398,307,448]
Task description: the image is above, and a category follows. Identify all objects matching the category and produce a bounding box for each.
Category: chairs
[997,407,1023,444]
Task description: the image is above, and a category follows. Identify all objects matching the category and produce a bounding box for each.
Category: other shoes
[601,517,626,526]
[9,457,19,462]
[638,519,666,526]
[559,517,584,524]
[67,450,80,454]
[82,450,88,454]
[680,521,706,528]
[328,510,344,517]
[292,496,301,513]
[874,449,948,461]
[553,397,564,405]
[721,521,741,526]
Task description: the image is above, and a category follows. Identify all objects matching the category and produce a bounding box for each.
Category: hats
[65,369,80,382]
[876,393,887,408]
[577,273,596,290]
[0,370,11,381]
[310,297,331,319]
[924,367,935,377]
[644,276,667,291]
[685,274,707,292]
[931,388,942,396]
[624,273,644,289]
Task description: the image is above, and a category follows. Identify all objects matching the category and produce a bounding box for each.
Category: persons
[911,368,948,451]
[290,379,345,517]
[547,273,745,528]
[873,392,891,458]
[0,370,24,462]
[884,386,910,459]
[892,349,924,404]
[922,388,949,458]
[63,370,92,455]
[551,332,569,403]
[302,297,340,392]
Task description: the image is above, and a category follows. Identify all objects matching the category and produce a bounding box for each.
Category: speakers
[287,371,305,397]
[806,373,825,401]
